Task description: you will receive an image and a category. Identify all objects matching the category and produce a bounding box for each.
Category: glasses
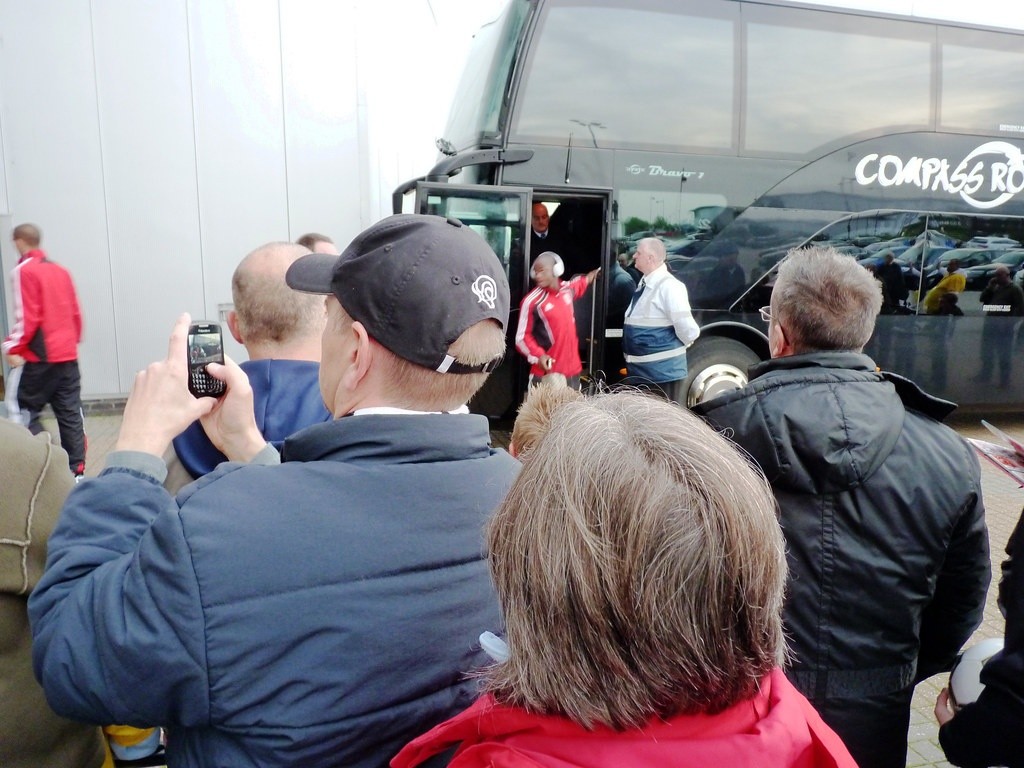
[758,304,790,345]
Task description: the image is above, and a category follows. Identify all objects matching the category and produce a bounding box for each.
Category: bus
[390,1,1024,420]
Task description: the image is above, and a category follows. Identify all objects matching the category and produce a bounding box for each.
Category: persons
[932,508,1024,768]
[689,247,991,768]
[505,386,583,461]
[170,234,340,475]
[607,245,1023,395]
[523,201,575,277]
[620,239,699,403]
[28,213,526,767]
[0,224,88,479]
[390,394,862,768]
[0,419,118,768]
[515,251,599,397]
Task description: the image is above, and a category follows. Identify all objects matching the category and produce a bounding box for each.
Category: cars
[622,233,1024,315]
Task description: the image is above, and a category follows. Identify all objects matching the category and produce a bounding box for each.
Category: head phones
[530,251,565,279]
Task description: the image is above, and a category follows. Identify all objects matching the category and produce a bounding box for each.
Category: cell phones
[187,321,227,400]
[546,358,552,368]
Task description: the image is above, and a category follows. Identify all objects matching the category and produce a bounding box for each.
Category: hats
[285,214,511,376]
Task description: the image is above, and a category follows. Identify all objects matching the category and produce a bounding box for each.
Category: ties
[540,233,546,239]
